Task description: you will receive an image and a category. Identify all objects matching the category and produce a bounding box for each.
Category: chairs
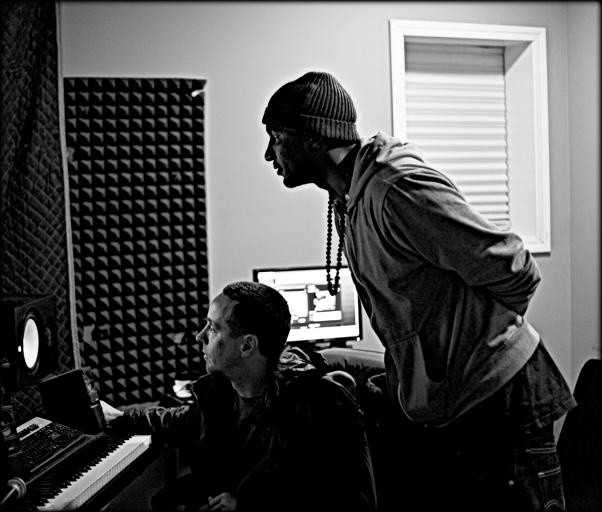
[556,359,602,511]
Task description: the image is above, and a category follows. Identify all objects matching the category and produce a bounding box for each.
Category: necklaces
[325,177,353,296]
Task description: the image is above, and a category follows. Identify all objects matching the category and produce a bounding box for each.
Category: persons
[259,73,579,510]
[98,281,379,510]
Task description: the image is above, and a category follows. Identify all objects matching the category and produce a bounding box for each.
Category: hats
[261,69,362,143]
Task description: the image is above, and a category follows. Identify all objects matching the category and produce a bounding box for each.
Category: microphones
[0,474,29,512]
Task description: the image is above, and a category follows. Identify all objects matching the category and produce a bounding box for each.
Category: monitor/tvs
[251,265,365,362]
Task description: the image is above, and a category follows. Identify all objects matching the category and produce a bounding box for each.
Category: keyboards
[9,414,86,473]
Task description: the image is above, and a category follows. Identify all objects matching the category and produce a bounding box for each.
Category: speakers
[1,290,61,392]
[37,365,108,434]
